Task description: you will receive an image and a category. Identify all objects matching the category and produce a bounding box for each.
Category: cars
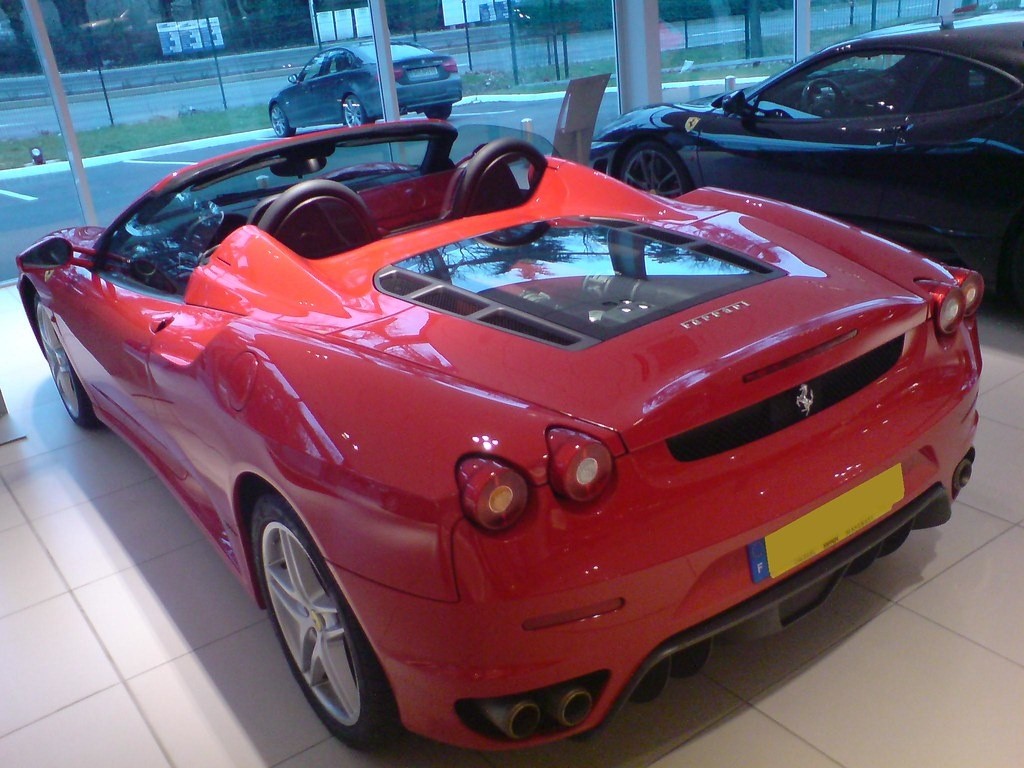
[588,6,1024,315]
[266,41,464,137]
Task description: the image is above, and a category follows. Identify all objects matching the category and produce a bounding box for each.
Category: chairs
[335,55,349,71]
[439,137,548,224]
[258,180,381,259]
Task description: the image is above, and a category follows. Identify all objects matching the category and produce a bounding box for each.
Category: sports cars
[17,113,984,750]
